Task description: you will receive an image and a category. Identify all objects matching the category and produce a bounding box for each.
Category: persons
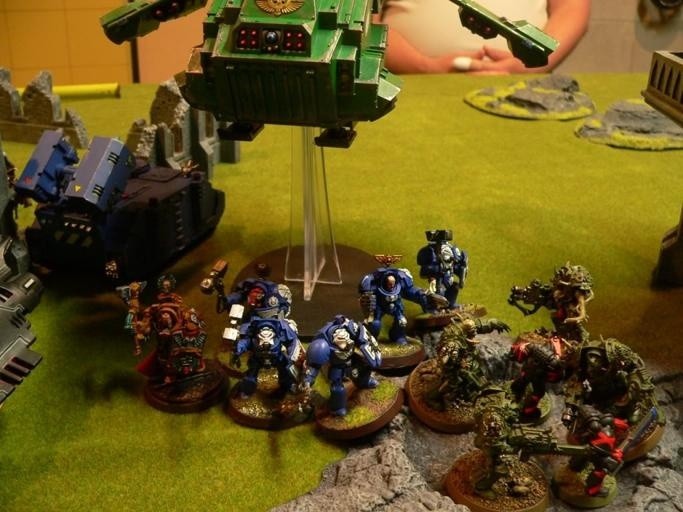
[368,0,592,77]
